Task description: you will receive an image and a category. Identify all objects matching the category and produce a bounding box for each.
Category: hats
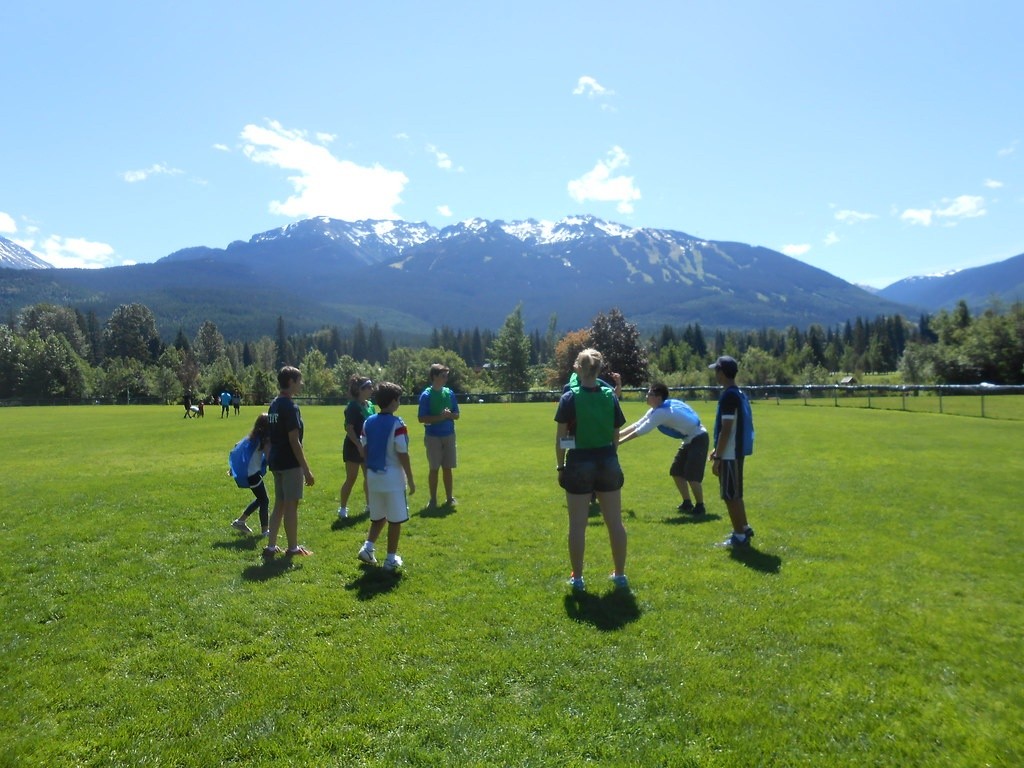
[709,356,738,375]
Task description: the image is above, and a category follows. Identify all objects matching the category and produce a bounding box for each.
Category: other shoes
[365,505,370,511]
[428,499,437,508]
[339,510,348,518]
[678,502,695,509]
[691,504,705,512]
[448,497,455,505]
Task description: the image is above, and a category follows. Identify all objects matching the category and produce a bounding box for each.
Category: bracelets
[557,467,565,471]
[714,457,722,461]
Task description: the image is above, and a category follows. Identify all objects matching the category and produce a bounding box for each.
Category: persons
[357,383,415,567]
[418,364,459,507]
[707,355,754,547]
[184,390,204,418]
[619,382,709,512]
[211,388,241,418]
[227,413,282,538]
[260,366,315,555]
[339,374,375,518]
[554,349,628,583]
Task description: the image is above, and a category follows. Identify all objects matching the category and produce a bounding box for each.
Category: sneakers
[231,519,252,533]
[730,528,754,537]
[286,547,313,557]
[383,559,402,570]
[569,577,583,586]
[263,532,282,538]
[609,572,626,584]
[722,536,749,546]
[264,547,285,556]
[357,547,377,564]
